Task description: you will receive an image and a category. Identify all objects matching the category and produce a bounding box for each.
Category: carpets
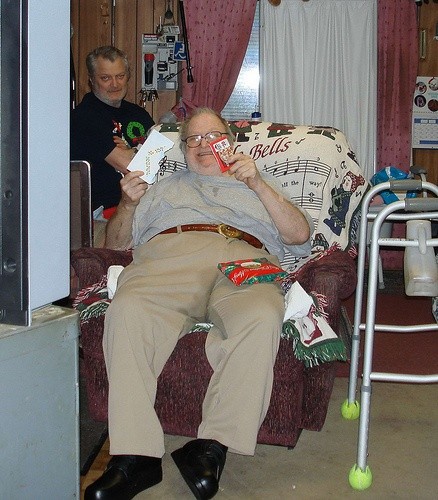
[335,288,438,384]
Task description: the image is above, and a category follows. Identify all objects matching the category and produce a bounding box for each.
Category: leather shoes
[170,439,228,499]
[84,452,163,500]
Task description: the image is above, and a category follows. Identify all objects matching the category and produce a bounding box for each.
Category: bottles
[251,112,262,121]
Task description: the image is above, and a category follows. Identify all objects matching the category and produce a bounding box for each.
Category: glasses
[182,131,229,148]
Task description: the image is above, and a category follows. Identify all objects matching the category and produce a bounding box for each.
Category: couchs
[70,120,372,449]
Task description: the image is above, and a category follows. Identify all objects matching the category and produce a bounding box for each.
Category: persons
[83,106,315,500]
[71,46,156,226]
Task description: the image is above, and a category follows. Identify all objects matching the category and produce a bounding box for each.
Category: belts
[156,223,271,254]
